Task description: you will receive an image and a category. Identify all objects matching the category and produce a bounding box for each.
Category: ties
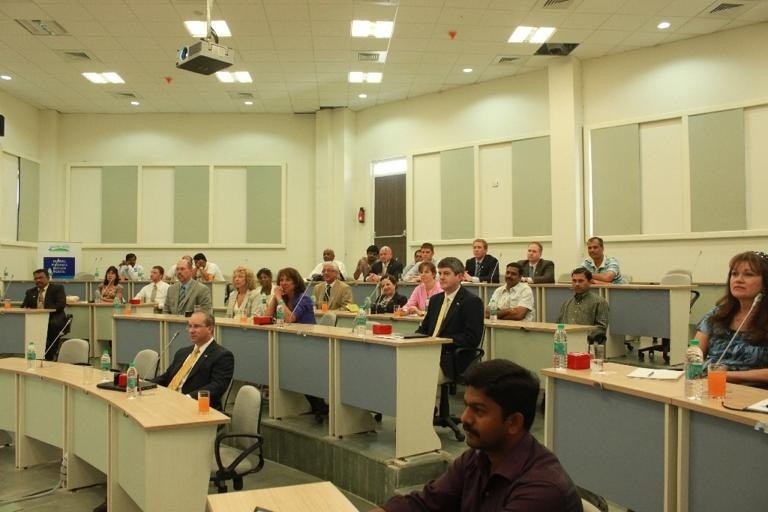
[149,285,157,303]
[167,347,208,393]
[382,262,387,276]
[37,287,46,309]
[475,262,479,276]
[178,285,186,305]
[323,283,332,305]
[431,298,449,340]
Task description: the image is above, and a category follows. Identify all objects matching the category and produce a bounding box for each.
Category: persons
[118,253,146,281]
[20,269,71,361]
[403,243,441,283]
[164,255,193,280]
[313,261,353,311]
[244,268,280,319]
[371,274,408,314]
[397,261,445,316]
[575,237,622,287]
[265,268,317,324]
[193,253,225,281]
[400,250,424,278]
[96,266,124,304]
[144,311,235,432]
[306,248,348,281]
[367,359,584,512]
[226,265,259,319]
[364,246,403,282]
[354,245,379,282]
[692,251,767,389]
[556,268,609,345]
[133,266,171,314]
[163,259,210,315]
[516,242,555,284]
[463,239,500,283]
[485,263,536,322]
[415,256,486,417]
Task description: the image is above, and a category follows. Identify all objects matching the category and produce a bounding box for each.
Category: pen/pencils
[647,370,656,379]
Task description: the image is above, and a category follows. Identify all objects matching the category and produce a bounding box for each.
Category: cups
[240,309,247,323]
[393,304,401,316]
[197,390,211,413]
[120,274,126,281]
[4,298,11,310]
[590,344,605,372]
[707,363,728,396]
[321,301,328,312]
[124,303,132,315]
[208,274,214,280]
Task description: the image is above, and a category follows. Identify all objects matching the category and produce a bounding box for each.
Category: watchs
[197,266,205,270]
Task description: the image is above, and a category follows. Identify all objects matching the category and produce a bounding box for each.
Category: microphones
[138,328,187,396]
[39,315,84,365]
[489,251,506,282]
[0,275,16,301]
[102,275,115,296]
[712,291,766,371]
[289,279,318,322]
[368,280,380,300]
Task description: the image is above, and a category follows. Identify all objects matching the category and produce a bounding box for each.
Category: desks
[204,480,360,512]
[110,312,454,461]
[2,278,228,317]
[315,308,597,395]
[228,275,698,372]
[539,360,768,512]
[0,308,56,360]
[0,357,231,512]
[2,298,159,361]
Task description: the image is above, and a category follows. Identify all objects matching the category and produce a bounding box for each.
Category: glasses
[321,268,336,273]
[34,276,45,280]
[185,324,212,330]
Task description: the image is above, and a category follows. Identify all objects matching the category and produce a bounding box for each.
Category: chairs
[46,313,73,362]
[576,484,608,512]
[433,348,485,442]
[210,385,264,494]
[636,270,706,362]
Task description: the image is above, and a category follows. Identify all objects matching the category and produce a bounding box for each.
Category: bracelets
[277,300,285,304]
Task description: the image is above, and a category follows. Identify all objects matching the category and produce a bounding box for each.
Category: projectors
[176,3,235,77]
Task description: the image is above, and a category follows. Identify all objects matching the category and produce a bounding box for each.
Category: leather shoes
[93,501,107,512]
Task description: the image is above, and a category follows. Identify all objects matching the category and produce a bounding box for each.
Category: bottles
[26,341,36,370]
[138,267,142,281]
[683,339,704,400]
[112,296,121,316]
[100,350,111,372]
[490,300,497,325]
[553,323,568,373]
[94,267,99,281]
[3,266,9,279]
[47,267,53,280]
[126,362,138,400]
[355,307,367,337]
[260,291,268,316]
[276,300,285,328]
